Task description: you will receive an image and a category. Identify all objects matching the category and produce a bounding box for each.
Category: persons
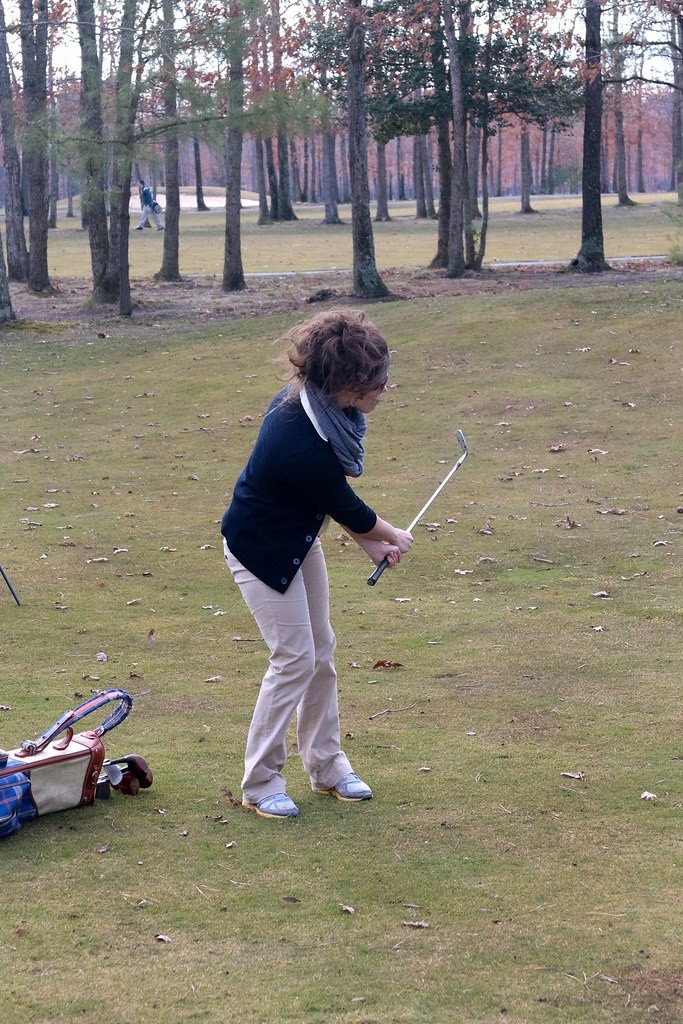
[132,180,165,231]
[219,307,413,817]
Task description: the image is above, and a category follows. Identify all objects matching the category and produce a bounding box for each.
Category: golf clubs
[367,430,469,585]
[95,755,152,799]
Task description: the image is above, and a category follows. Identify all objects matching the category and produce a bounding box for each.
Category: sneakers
[316,771,372,802]
[243,790,299,819]
